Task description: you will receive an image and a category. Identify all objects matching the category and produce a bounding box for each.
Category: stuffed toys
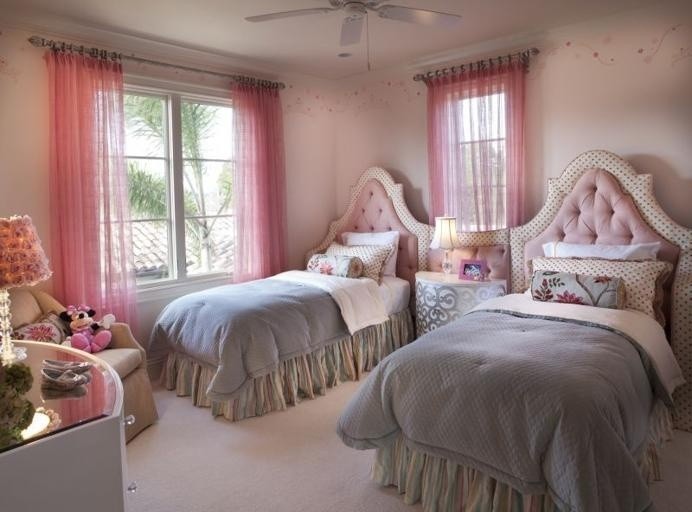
[58,303,116,355]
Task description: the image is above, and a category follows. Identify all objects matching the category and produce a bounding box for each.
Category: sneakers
[41,359,93,374]
[40,368,87,392]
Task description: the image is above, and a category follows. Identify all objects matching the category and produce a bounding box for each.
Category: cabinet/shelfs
[0,403,137,512]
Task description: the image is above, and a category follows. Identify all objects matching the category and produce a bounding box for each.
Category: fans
[244,0,462,47]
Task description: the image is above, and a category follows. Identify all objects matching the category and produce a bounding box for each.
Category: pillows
[10,310,69,345]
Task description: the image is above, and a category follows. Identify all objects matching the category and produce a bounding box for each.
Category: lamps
[1,215,53,366]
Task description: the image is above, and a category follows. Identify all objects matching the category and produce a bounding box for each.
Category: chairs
[8,286,159,445]
